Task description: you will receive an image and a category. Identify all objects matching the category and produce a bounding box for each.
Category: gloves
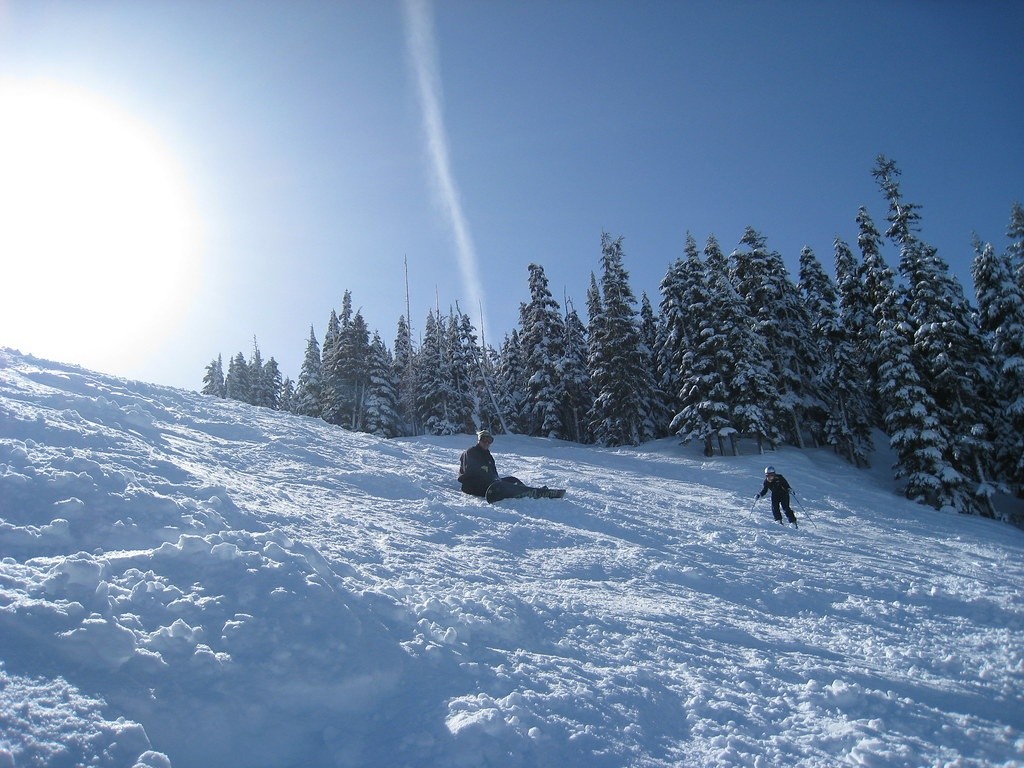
[790,489,797,496]
[753,494,760,499]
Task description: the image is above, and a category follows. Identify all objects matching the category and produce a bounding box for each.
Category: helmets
[765,466,775,473]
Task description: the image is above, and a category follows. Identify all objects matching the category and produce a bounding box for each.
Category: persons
[457,429,549,498]
[754,465,798,529]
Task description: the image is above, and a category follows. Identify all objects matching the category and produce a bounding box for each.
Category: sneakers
[789,522,797,530]
[775,519,782,524]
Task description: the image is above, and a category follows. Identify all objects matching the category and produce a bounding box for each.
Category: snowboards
[485,480,566,504]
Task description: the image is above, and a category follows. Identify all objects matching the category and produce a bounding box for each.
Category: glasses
[765,474,774,477]
[479,436,494,443]
